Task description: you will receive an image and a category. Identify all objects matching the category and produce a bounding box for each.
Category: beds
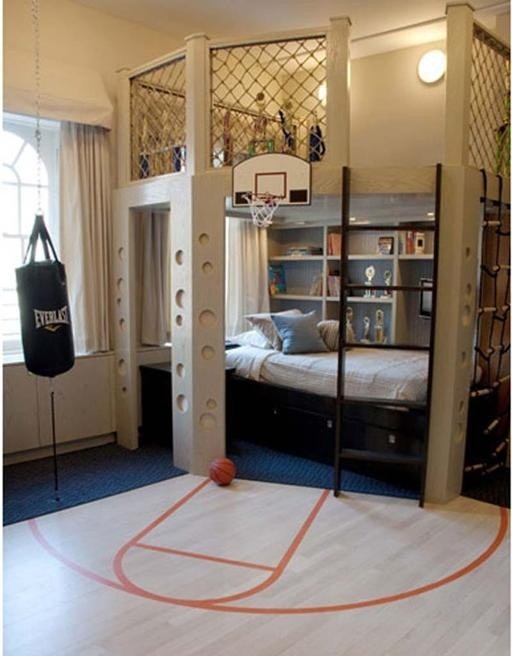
[225,325,484,499]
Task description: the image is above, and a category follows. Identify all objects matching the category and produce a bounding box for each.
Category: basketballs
[208,457,236,485]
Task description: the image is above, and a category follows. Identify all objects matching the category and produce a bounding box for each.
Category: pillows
[244,307,303,351]
[270,309,331,355]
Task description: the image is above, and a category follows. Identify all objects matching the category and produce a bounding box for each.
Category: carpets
[4,434,494,529]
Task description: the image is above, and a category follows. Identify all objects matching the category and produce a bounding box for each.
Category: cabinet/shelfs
[256,216,436,346]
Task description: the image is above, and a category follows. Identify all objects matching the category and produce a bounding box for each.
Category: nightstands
[139,360,238,458]
